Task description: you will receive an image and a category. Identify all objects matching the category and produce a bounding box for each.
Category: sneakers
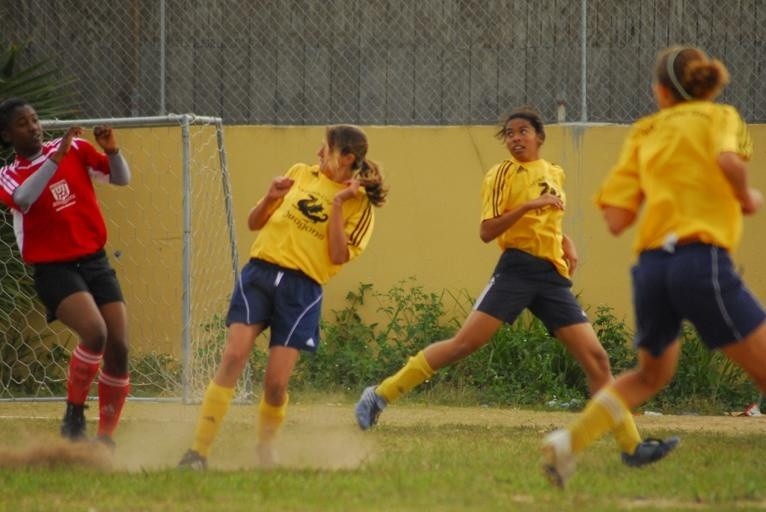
[354,384,387,432]
[178,446,208,475]
[620,434,679,468]
[60,404,87,439]
[539,427,577,491]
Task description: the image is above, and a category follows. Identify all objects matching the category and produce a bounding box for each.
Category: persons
[176,127,390,471]
[541,46,766,490]
[356,113,680,468]
[0,98,131,456]
